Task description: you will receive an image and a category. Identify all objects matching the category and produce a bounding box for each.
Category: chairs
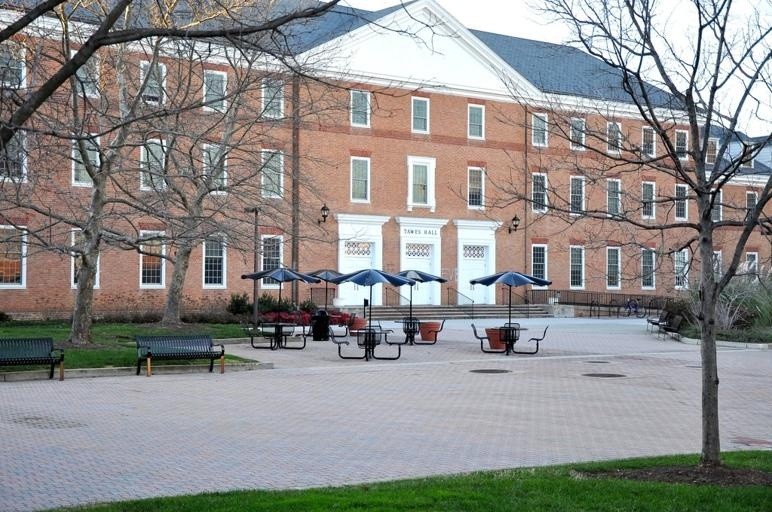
[648,311,684,342]
[471,322,549,355]
[239,312,445,361]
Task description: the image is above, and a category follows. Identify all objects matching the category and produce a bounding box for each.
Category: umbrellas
[334,269,415,348]
[307,268,344,332]
[240,265,320,345]
[469,269,552,351]
[394,270,447,338]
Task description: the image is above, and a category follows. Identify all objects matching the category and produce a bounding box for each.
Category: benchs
[0,337,63,381]
[133,334,225,377]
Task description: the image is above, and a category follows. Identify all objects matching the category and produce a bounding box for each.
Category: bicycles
[617,295,646,320]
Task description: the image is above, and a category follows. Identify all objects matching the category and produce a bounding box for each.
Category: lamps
[318,203,329,224]
[509,213,521,233]
